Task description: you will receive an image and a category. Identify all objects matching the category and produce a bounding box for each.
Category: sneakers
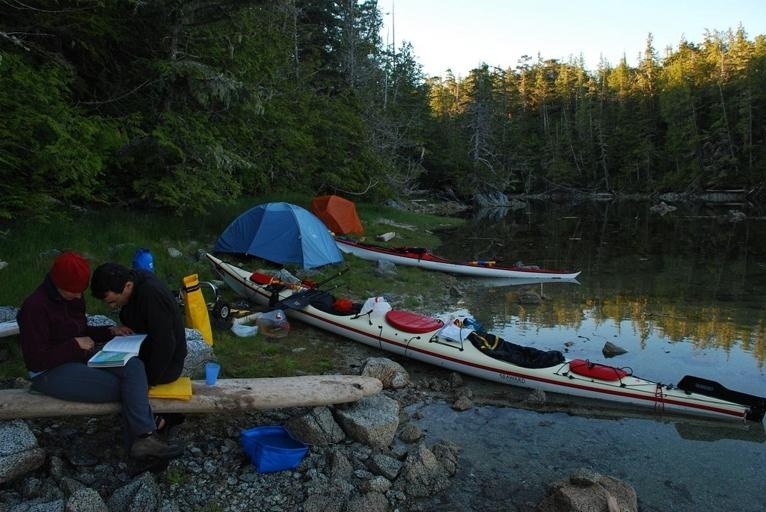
[130,434,183,458]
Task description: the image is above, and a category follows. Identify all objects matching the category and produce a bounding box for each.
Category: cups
[204,363,221,385]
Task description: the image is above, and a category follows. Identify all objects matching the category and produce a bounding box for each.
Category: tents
[311,195,364,233]
[212,201,344,271]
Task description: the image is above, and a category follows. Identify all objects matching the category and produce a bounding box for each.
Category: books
[86,334,148,369]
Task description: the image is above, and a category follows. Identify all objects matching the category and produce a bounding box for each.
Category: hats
[51,252,90,293]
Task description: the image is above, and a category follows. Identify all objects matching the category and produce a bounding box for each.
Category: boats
[200,248,766,427]
[335,234,581,280]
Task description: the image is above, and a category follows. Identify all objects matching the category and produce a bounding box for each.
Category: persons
[90,262,188,386]
[17,251,185,479]
